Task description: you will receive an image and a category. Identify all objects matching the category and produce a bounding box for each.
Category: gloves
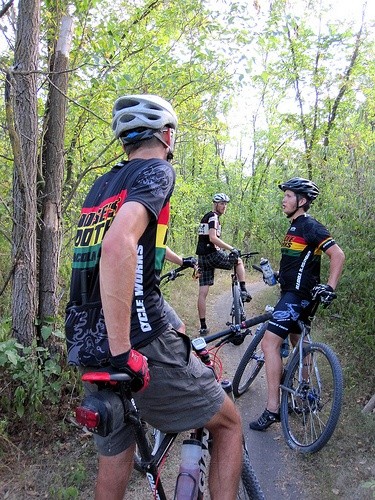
[118,349,151,394]
[311,284,333,301]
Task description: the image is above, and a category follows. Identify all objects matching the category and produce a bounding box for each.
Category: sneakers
[249,408,281,431]
[241,291,251,299]
[200,327,210,336]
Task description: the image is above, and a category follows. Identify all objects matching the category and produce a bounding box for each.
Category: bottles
[280,336,290,357]
[259,257,277,287]
[175,432,202,500]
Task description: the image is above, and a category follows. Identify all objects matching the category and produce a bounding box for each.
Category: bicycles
[231,264,344,454]
[120,259,192,474]
[225,249,259,326]
[75,311,274,500]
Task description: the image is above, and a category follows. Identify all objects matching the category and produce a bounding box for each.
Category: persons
[249,178,345,431]
[195,192,252,336]
[65,94,243,500]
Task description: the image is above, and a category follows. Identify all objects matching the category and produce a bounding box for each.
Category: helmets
[212,193,230,203]
[278,176,320,200]
[111,94,178,145]
